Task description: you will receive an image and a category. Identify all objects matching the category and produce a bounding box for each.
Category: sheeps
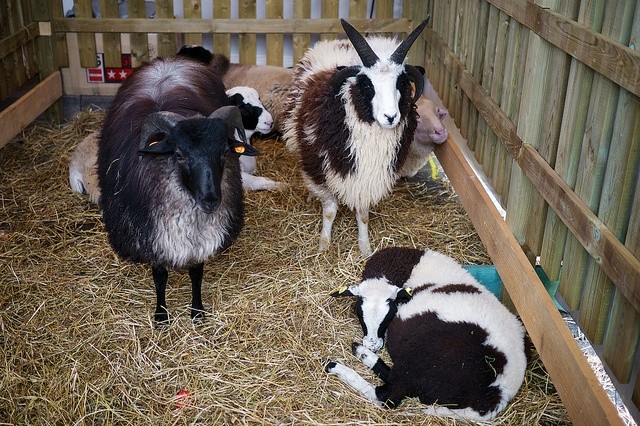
[95,53,257,323]
[68,86,283,203]
[283,15,430,258]
[400,80,448,176]
[224,60,295,135]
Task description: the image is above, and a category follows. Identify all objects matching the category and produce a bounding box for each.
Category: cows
[327,247,533,423]
